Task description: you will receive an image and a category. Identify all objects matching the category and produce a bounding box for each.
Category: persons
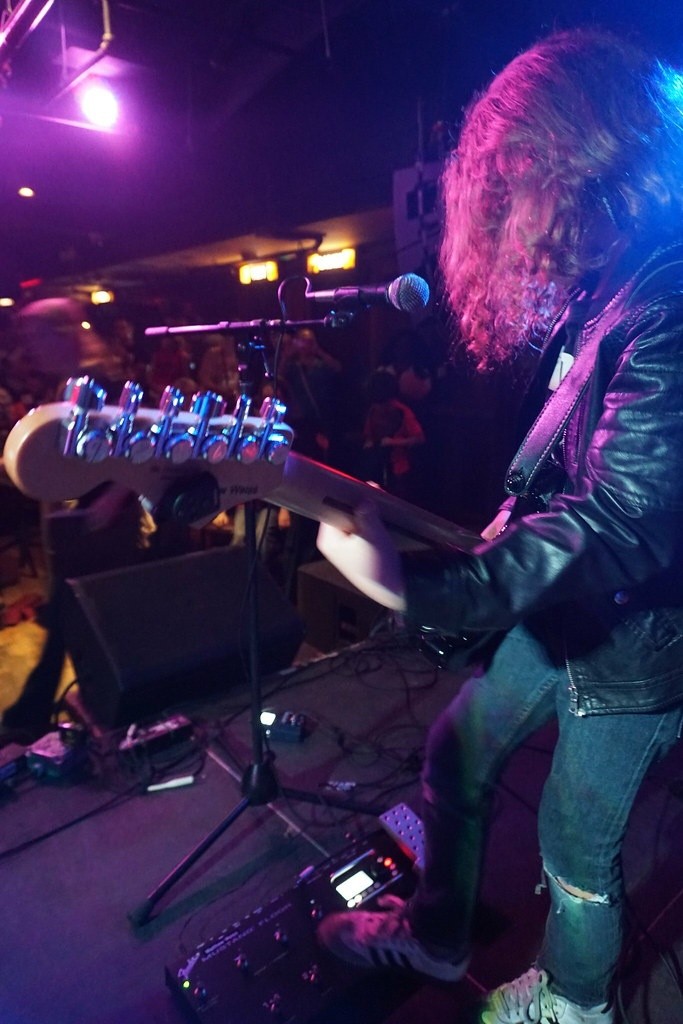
[0,315,472,601]
[309,24,682,1024]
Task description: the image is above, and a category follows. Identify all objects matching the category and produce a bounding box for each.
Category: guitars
[0,372,549,676]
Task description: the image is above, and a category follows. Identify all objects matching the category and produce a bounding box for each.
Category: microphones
[306,274,429,316]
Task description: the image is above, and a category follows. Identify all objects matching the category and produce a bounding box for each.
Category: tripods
[121,312,391,930]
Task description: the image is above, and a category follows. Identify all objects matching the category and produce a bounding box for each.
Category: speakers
[61,540,301,735]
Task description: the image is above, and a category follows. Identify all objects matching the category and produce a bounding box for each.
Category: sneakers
[479,967,615,1024]
[318,895,472,982]
